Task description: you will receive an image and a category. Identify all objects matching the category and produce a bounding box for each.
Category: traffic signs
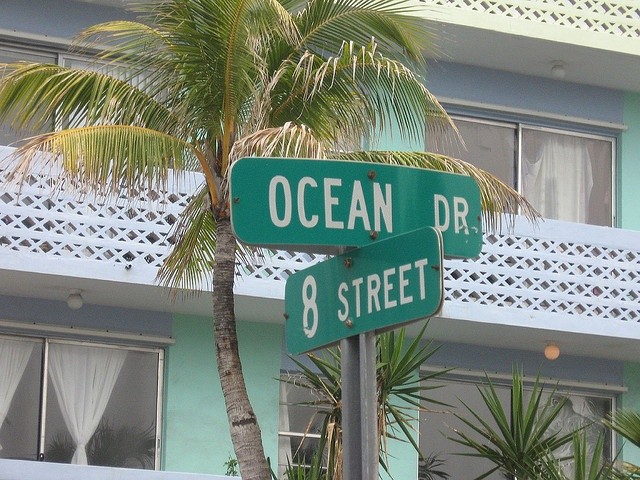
[284,226,444,355]
[228,157,483,260]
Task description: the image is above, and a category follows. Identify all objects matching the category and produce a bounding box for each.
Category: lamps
[66,288,85,312]
[544,340,561,360]
[549,59,568,80]
